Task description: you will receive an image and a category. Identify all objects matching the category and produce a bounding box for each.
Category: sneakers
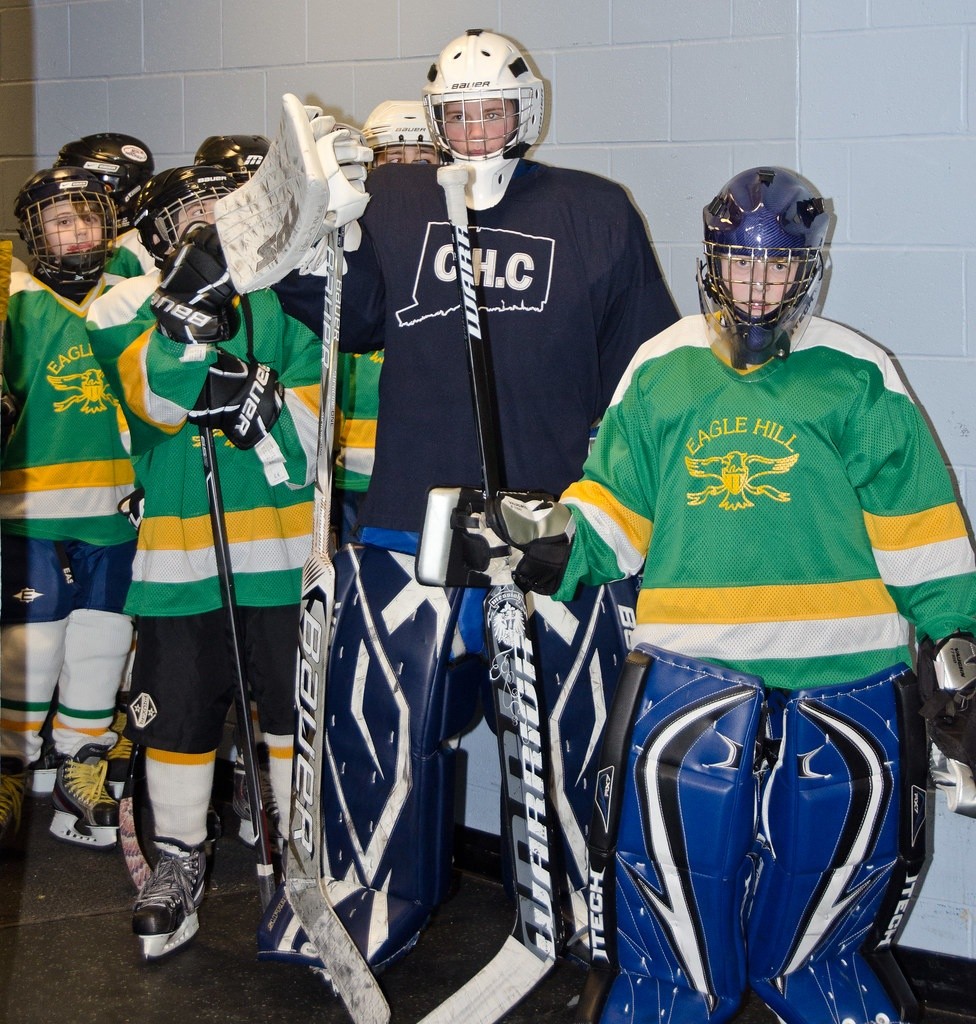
[2,736,119,845]
[132,836,208,957]
[106,692,137,799]
[230,729,280,845]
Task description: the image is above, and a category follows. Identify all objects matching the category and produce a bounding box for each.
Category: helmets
[421,30,545,212]
[13,167,117,293]
[696,167,828,370]
[52,133,154,238]
[362,100,437,174]
[194,135,271,189]
[133,165,238,268]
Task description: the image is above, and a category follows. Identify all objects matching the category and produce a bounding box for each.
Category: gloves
[187,344,285,452]
[920,637,975,779]
[151,225,242,345]
[449,491,571,597]
[117,488,146,534]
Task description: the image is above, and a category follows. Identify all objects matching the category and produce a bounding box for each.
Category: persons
[193,135,283,855]
[331,99,448,551]
[86,166,338,960]
[252,28,684,972]
[464,167,976,1024]
[0,167,135,847]
[24,133,154,797]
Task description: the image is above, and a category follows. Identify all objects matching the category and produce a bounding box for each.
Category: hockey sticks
[280,225,402,1024]
[407,161,556,1024]
[200,423,279,913]
[115,742,154,891]
